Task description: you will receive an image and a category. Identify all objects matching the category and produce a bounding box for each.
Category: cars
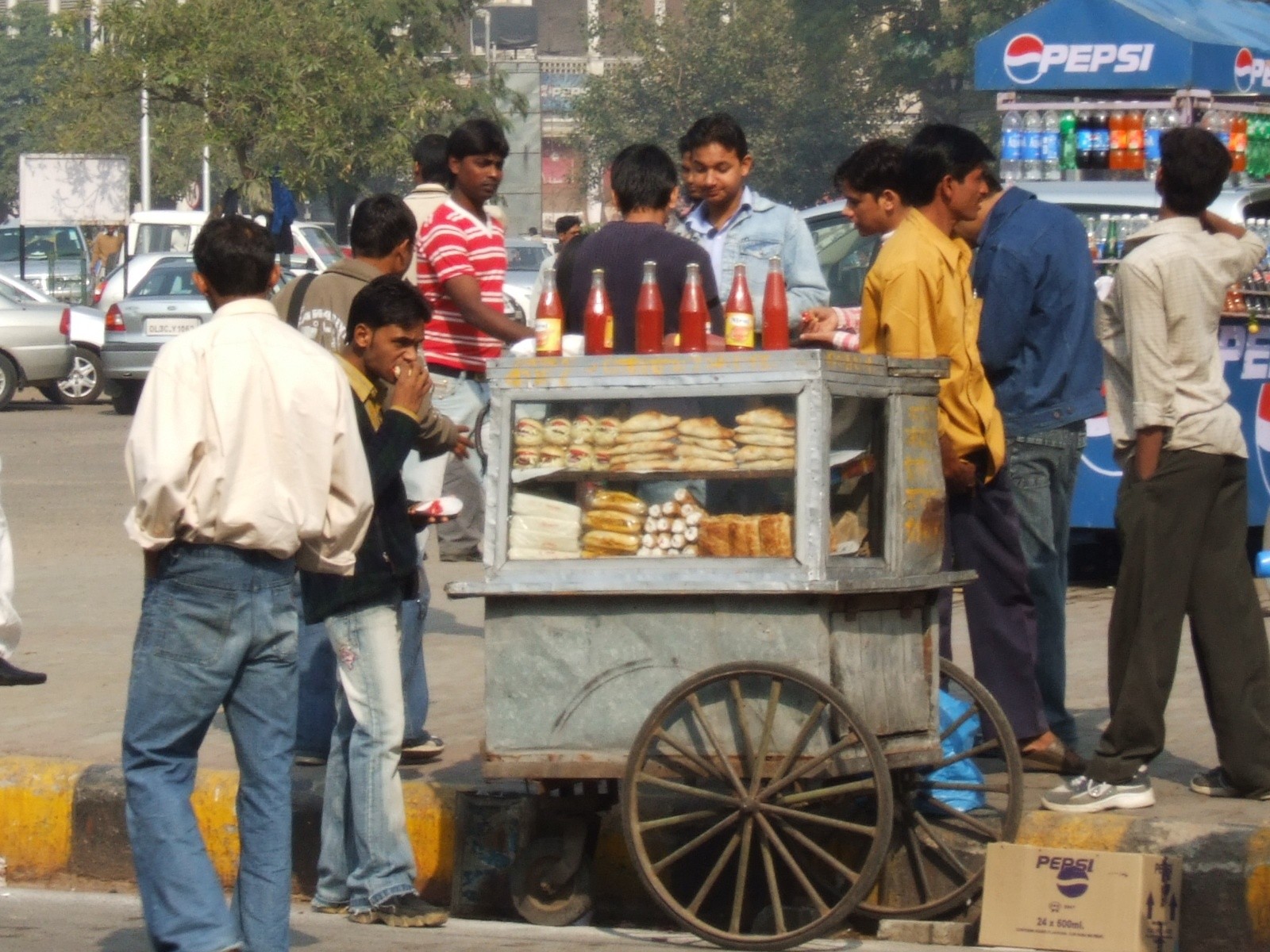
[503,236,560,331]
[94,210,351,414]
[0,216,93,307]
[0,279,75,412]
[0,266,105,405]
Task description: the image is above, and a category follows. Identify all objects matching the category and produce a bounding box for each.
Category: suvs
[800,179,1270,306]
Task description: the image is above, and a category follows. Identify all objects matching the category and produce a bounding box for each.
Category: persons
[402,118,538,563]
[528,216,582,330]
[795,122,1105,776]
[122,187,374,952]
[299,271,460,930]
[268,193,477,766]
[556,144,725,355]
[403,133,457,288]
[1,498,46,686]
[671,113,831,333]
[1040,126,1270,816]
[89,226,122,300]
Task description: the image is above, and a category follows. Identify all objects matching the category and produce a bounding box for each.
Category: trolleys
[443,338,1026,952]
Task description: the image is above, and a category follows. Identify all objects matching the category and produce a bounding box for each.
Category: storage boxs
[978,840,1184,952]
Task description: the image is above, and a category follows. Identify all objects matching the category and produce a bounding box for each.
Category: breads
[506,489,794,560]
[393,365,412,378]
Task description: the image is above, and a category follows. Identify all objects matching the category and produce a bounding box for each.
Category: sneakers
[1190,767,1269,799]
[317,903,350,915]
[1041,764,1154,812]
[349,893,448,927]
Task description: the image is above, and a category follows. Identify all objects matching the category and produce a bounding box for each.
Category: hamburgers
[513,416,621,472]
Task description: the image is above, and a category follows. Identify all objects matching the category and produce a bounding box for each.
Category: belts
[427,364,486,382]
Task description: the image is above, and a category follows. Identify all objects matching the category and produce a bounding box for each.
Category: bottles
[584,268,614,355]
[535,267,564,357]
[762,256,790,349]
[635,262,665,355]
[725,263,755,352]
[1083,214,1270,314]
[1000,100,1270,180]
[679,263,709,352]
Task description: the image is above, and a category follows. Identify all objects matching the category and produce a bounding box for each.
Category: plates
[416,496,463,517]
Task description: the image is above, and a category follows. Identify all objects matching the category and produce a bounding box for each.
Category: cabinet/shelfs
[491,382,898,572]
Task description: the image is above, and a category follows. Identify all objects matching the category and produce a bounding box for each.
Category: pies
[608,406,797,473]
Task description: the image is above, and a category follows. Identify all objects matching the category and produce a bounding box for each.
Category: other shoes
[401,736,445,762]
[294,749,328,765]
[441,550,480,561]
[974,733,1002,758]
[0,658,46,685]
[1020,740,1086,776]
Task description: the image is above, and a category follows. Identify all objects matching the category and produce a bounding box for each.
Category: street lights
[473,7,492,96]
